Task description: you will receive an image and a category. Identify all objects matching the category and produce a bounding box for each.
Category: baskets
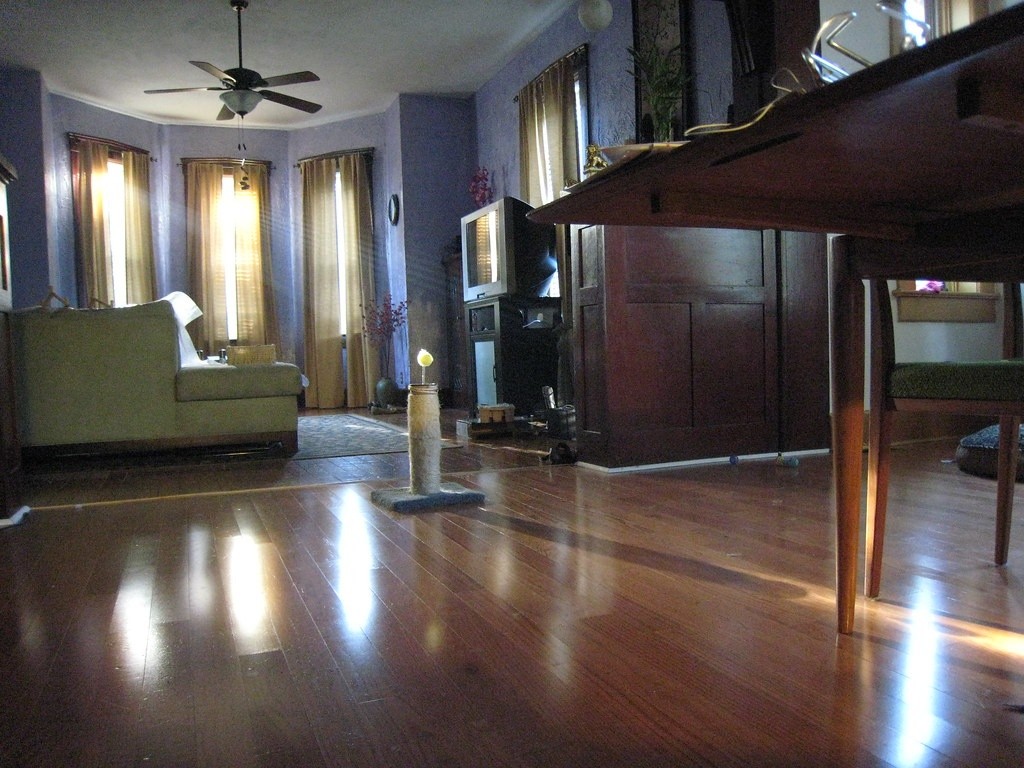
[226,344,276,366]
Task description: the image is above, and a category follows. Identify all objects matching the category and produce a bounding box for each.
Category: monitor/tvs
[460,196,558,298]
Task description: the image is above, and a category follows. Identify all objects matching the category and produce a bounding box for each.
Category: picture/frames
[632,0,690,144]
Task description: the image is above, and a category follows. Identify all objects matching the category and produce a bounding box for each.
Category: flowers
[468,166,494,210]
[359,294,412,377]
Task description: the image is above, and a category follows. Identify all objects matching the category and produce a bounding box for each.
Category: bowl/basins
[598,140,691,164]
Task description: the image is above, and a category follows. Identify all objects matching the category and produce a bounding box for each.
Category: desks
[524,1,1024,633]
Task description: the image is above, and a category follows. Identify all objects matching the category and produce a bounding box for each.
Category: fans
[144,0,322,120]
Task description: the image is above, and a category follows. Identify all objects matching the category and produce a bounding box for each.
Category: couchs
[7,301,303,465]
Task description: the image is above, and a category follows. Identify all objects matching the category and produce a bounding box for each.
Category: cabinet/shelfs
[463,295,561,418]
[576,224,779,470]
[440,237,469,406]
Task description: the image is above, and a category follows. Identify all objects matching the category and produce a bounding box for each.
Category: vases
[376,377,400,409]
[647,92,674,143]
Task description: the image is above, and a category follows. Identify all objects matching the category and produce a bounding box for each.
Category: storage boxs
[455,404,531,437]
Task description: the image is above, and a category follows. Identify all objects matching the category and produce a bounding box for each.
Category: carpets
[186,414,464,464]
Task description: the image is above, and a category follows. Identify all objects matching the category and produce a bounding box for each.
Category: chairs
[864,280,1024,604]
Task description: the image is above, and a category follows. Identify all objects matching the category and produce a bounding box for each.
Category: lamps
[416,348,433,384]
[219,90,264,191]
